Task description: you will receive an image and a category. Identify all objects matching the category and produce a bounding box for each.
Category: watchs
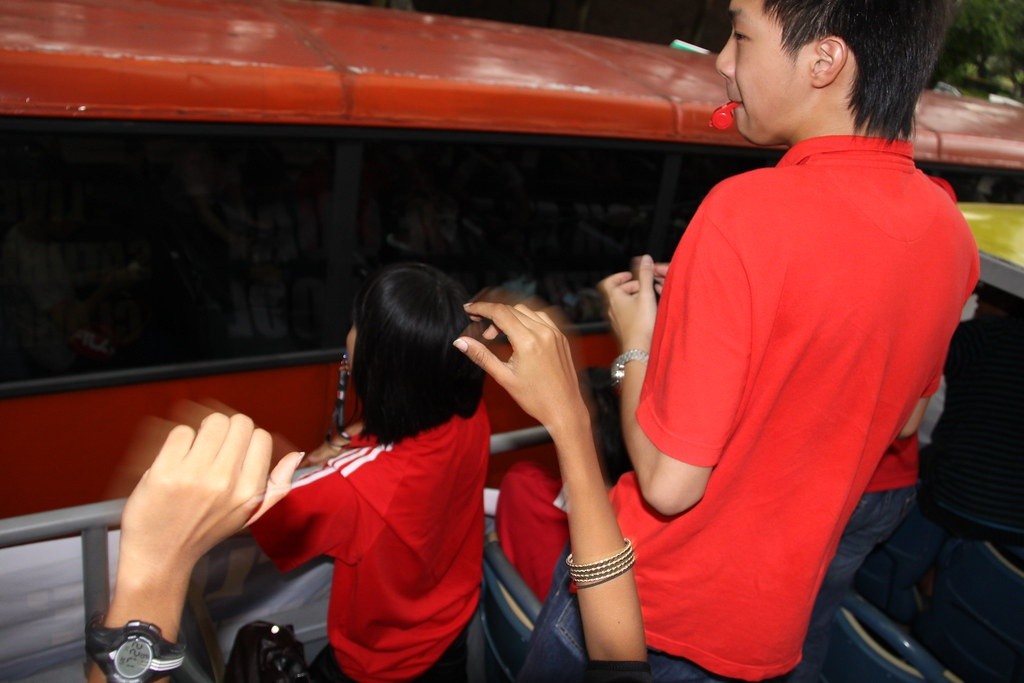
[611,349,650,386]
[83,610,187,683]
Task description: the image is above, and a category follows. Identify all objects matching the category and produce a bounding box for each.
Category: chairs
[468,526,1023,683]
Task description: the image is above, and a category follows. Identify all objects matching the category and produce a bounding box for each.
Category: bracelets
[565,536,636,582]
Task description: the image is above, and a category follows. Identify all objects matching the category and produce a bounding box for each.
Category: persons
[240,263,491,683]
[93,303,650,683]
[495,0,1024,683]
